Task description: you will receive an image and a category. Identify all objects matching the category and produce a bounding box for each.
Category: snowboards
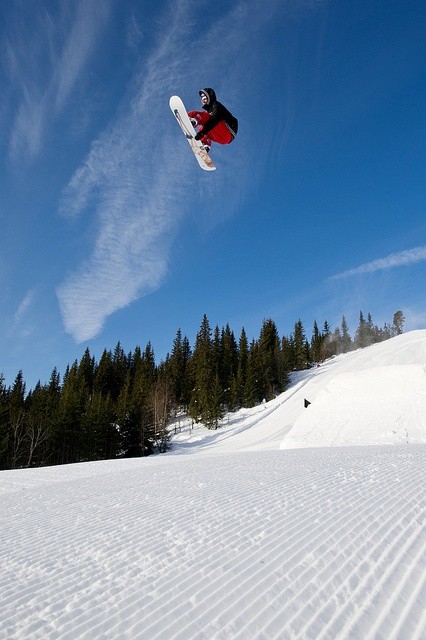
[169,96,217,171]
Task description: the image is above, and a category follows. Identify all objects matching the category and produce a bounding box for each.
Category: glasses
[200,96,204,100]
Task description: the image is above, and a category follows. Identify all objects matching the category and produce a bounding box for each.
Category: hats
[200,92,208,102]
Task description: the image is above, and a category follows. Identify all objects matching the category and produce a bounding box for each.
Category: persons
[187,87,240,153]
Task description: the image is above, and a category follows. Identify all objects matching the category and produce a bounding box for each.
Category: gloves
[195,131,205,140]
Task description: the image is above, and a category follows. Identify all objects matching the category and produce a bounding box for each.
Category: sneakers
[191,118,197,128]
[202,142,211,152]
